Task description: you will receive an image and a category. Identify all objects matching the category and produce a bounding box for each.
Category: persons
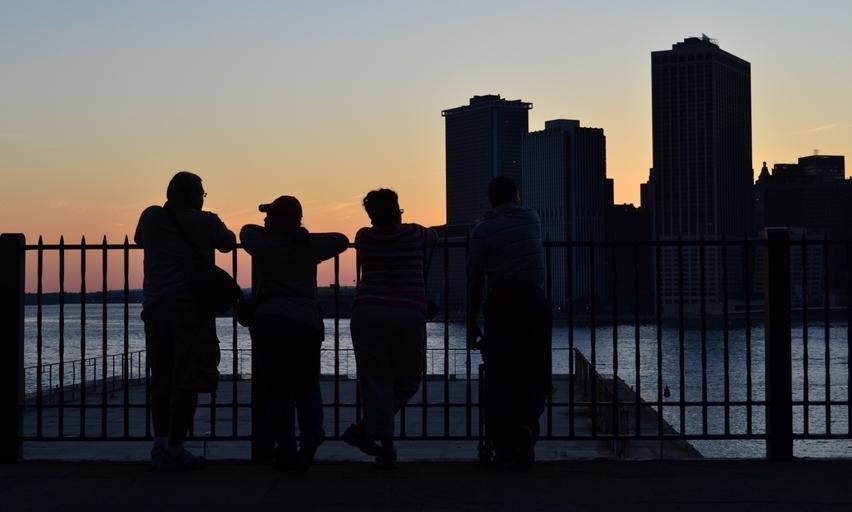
[340,187,439,468]
[460,174,555,476]
[133,169,238,472]
[238,194,351,472]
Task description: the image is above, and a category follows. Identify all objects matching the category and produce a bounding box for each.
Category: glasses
[200,192,208,197]
[397,208,404,215]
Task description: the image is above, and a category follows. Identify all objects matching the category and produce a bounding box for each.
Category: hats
[258,195,303,217]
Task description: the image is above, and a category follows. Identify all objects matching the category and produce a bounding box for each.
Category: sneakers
[515,426,536,472]
[340,423,398,472]
[274,438,318,475]
[149,438,207,475]
